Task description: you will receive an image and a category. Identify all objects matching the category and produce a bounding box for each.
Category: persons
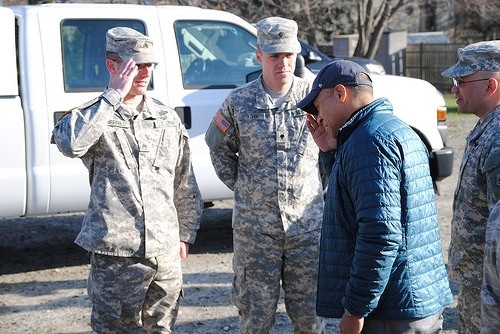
[51,26,204,334]
[480,198,500,334]
[205,16,324,334]
[295,58,453,334]
[441,40,500,334]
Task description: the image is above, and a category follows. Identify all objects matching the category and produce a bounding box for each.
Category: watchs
[343,307,357,317]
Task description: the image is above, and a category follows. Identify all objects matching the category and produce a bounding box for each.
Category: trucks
[0,4,456,219]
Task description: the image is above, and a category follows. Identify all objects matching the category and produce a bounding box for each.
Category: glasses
[111,59,152,69]
[452,78,491,87]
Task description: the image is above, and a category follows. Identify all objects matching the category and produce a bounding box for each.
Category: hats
[440,41,500,78]
[106,27,159,63]
[293,58,375,115]
[256,17,303,54]
